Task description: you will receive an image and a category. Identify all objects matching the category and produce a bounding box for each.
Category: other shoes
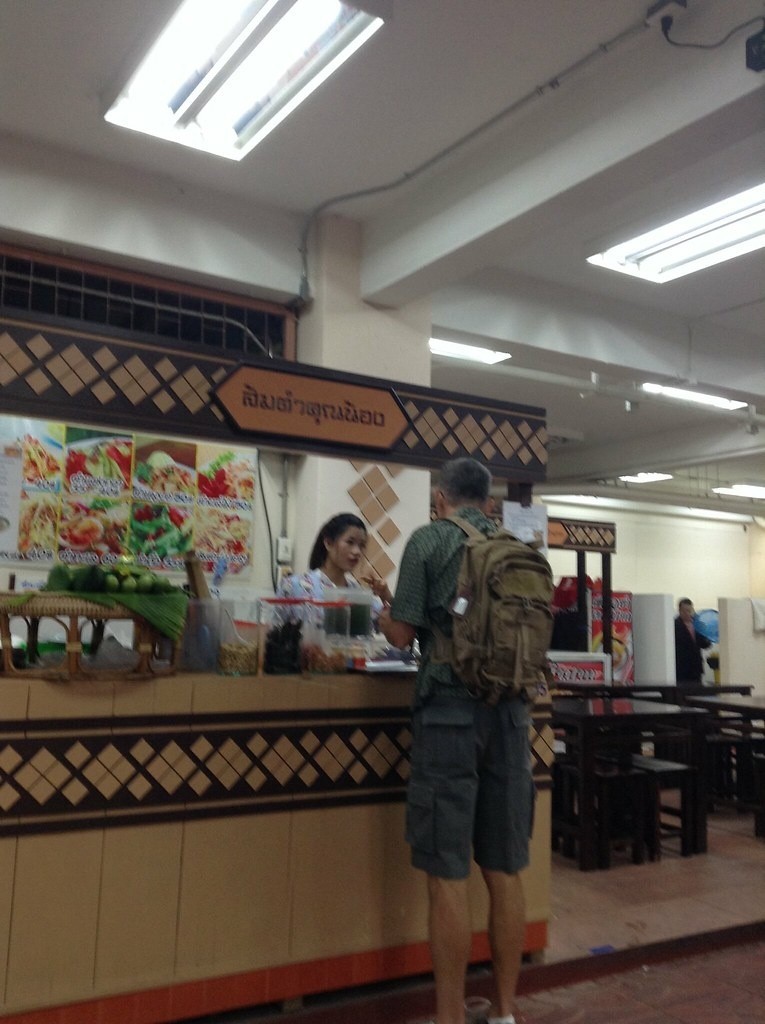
[462,997,515,1024]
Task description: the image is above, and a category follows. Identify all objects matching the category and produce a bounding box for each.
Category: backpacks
[421,515,554,703]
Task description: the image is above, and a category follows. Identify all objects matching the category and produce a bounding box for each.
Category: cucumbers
[47,563,172,593]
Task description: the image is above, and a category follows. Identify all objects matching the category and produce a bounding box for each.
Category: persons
[675,599,710,685]
[377,458,534,1024]
[278,514,382,632]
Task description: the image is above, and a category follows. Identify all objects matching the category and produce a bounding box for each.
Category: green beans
[127,502,192,558]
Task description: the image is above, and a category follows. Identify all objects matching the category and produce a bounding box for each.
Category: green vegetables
[88,462,152,509]
[206,451,235,477]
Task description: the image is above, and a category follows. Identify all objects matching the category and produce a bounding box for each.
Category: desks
[686,693,765,814]
[553,697,710,864]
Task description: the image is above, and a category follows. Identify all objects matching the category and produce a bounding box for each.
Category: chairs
[552,716,765,869]
